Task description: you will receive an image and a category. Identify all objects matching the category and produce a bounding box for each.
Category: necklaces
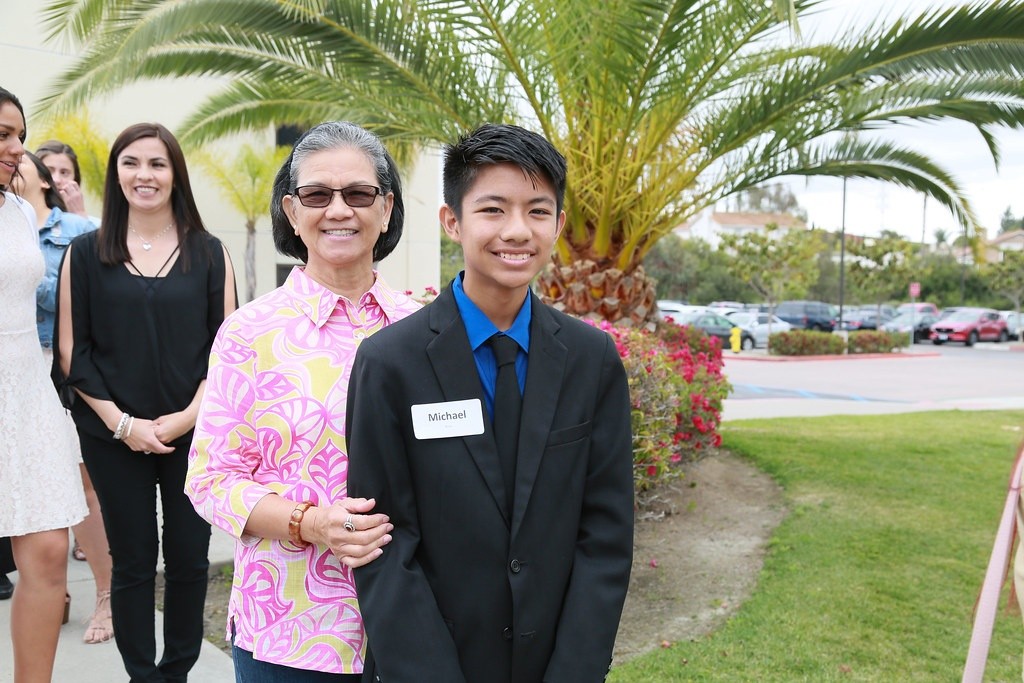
[128,222,177,252]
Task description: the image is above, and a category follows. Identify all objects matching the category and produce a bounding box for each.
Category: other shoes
[0,571,14,600]
[73,537,87,561]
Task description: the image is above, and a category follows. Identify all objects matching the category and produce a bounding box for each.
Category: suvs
[772,302,835,333]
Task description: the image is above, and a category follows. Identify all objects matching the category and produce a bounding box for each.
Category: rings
[144,451,151,454]
[343,514,356,533]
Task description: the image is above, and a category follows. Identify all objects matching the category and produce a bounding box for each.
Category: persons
[0,86,90,683]
[50,122,239,683]
[344,124,635,683]
[4,140,115,643]
[184,122,427,683]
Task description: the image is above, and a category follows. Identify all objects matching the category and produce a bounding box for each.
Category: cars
[680,314,754,354]
[657,301,1024,348]
[931,309,1008,346]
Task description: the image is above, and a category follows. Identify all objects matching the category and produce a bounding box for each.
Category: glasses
[291,185,385,207]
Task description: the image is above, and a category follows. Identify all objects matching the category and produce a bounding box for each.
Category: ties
[485,333,523,518]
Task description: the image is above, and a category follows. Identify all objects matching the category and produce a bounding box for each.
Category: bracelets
[112,412,134,441]
[288,500,315,548]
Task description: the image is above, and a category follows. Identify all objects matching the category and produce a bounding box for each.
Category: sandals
[62,593,71,624]
[81,589,115,644]
